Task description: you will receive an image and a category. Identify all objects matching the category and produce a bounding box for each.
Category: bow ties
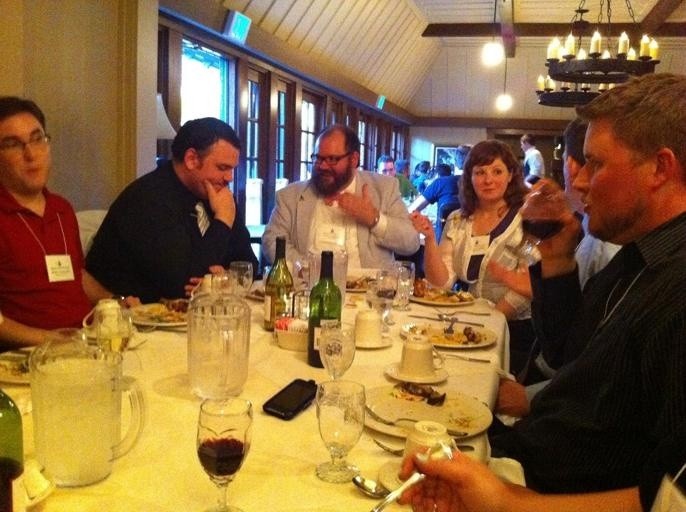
[324,192,340,204]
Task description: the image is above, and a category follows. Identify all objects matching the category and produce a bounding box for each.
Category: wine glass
[212,261,253,299]
[195,319,363,511]
[367,260,416,333]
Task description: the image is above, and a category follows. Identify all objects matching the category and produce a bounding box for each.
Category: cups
[293,242,348,306]
[189,294,249,399]
[399,335,445,377]
[31,347,145,487]
[82,299,122,333]
[399,421,461,478]
[355,308,381,345]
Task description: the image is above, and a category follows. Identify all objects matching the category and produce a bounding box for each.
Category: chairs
[73,207,107,253]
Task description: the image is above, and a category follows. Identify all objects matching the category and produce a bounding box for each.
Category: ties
[195,202,210,236]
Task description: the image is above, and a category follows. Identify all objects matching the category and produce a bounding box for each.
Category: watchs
[111,295,126,303]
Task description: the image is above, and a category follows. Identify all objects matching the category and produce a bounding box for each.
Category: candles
[578,48,586,60]
[582,83,590,88]
[609,82,614,89]
[628,47,636,60]
[640,34,649,56]
[619,31,628,53]
[599,82,607,89]
[547,39,558,58]
[602,49,610,59]
[538,76,545,90]
[562,81,570,87]
[565,32,574,55]
[590,31,599,52]
[649,40,659,60]
[546,75,552,88]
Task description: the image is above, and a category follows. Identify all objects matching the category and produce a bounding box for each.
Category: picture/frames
[433,145,463,176]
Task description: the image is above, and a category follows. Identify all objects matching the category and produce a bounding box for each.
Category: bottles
[0,389,23,512]
[262,235,341,367]
[409,191,414,203]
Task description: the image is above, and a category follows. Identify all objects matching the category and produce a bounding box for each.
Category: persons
[258,124,419,284]
[373,73,686,512]
[85,116,258,302]
[0,95,141,348]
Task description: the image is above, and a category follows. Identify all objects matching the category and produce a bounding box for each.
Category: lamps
[482,1,505,64]
[495,54,514,111]
[157,94,177,140]
[535,9,615,107]
[547,0,661,84]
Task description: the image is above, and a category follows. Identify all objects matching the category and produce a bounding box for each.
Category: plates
[131,302,187,327]
[351,361,493,440]
[247,285,265,301]
[346,289,367,292]
[0,351,28,385]
[401,295,496,348]
[355,336,392,348]
[86,333,127,345]
[379,462,403,494]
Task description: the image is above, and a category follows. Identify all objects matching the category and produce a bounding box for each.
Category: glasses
[0,136,51,150]
[311,153,350,163]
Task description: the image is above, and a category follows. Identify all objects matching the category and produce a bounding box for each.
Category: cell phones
[262,379,317,421]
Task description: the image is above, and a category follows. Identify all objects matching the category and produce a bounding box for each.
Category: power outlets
[0,289,527,512]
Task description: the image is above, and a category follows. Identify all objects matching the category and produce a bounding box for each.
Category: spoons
[352,475,389,497]
